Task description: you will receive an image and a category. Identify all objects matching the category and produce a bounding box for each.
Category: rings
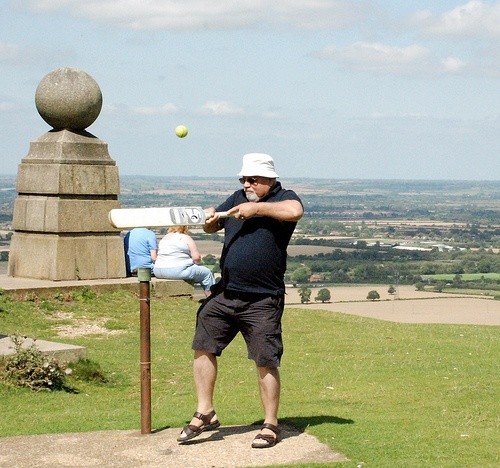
[242,217,243,219]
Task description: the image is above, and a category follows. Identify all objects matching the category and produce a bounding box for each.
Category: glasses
[238,177,260,184]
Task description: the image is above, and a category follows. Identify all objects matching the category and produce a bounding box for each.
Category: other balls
[174,125,188,138]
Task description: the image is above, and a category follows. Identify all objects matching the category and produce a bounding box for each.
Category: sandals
[251,422,281,449]
[176,410,221,442]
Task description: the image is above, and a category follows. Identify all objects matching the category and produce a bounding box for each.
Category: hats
[237,152,280,179]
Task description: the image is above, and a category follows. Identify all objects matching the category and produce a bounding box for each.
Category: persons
[153,226,215,296]
[124,227,158,277]
[177,152,304,448]
